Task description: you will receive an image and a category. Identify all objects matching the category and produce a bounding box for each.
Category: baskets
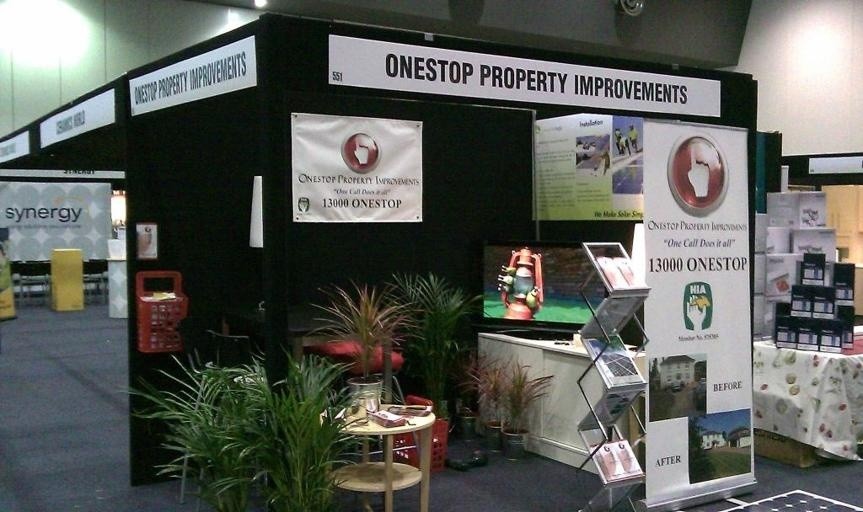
[392,394,449,475]
[135,270,190,353]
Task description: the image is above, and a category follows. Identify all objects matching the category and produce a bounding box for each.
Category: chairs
[179,328,270,512]
[9,257,108,313]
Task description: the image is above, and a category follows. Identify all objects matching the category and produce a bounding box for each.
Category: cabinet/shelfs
[476,333,645,475]
[574,240,652,512]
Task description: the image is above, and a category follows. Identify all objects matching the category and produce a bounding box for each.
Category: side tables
[319,403,437,512]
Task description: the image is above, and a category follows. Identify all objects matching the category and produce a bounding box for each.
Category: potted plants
[384,273,483,468]
[469,344,513,452]
[499,361,553,461]
[304,281,413,414]
[455,399,477,437]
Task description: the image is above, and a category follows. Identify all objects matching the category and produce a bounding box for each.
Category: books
[592,437,644,483]
[593,252,649,295]
[586,334,644,389]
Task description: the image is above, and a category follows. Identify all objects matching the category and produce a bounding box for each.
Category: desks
[752,325,863,462]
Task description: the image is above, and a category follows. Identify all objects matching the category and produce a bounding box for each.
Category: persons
[686,295,706,330]
[613,124,639,156]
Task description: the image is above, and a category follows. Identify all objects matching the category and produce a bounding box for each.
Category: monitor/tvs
[470,238,612,341]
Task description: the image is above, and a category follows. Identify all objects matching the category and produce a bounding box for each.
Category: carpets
[717,489,863,512]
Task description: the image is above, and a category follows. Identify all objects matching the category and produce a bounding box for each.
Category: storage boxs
[753,190,837,335]
[753,389,827,469]
